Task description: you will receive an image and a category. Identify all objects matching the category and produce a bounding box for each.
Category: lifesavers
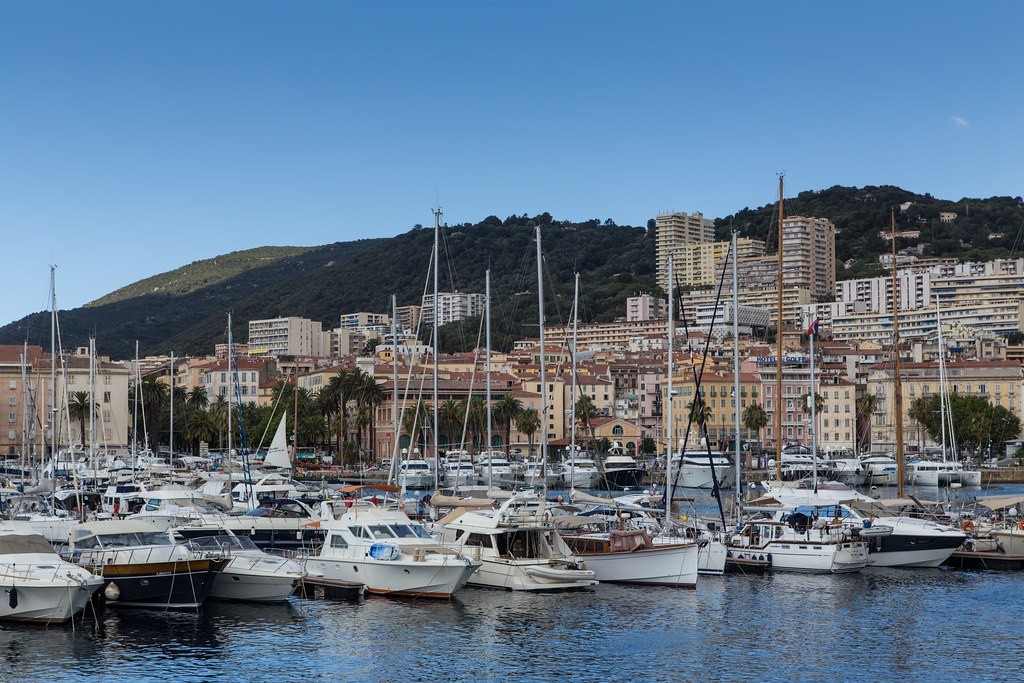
[1018,520,1024,530]
[962,520,975,533]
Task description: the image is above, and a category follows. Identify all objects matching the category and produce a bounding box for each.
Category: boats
[59,517,234,619]
[164,522,311,605]
[0,528,104,628]
[291,506,483,600]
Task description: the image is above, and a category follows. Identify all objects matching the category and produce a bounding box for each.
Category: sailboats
[0,171,1024,590]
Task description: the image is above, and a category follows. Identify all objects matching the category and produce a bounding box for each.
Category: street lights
[426,493,600,593]
